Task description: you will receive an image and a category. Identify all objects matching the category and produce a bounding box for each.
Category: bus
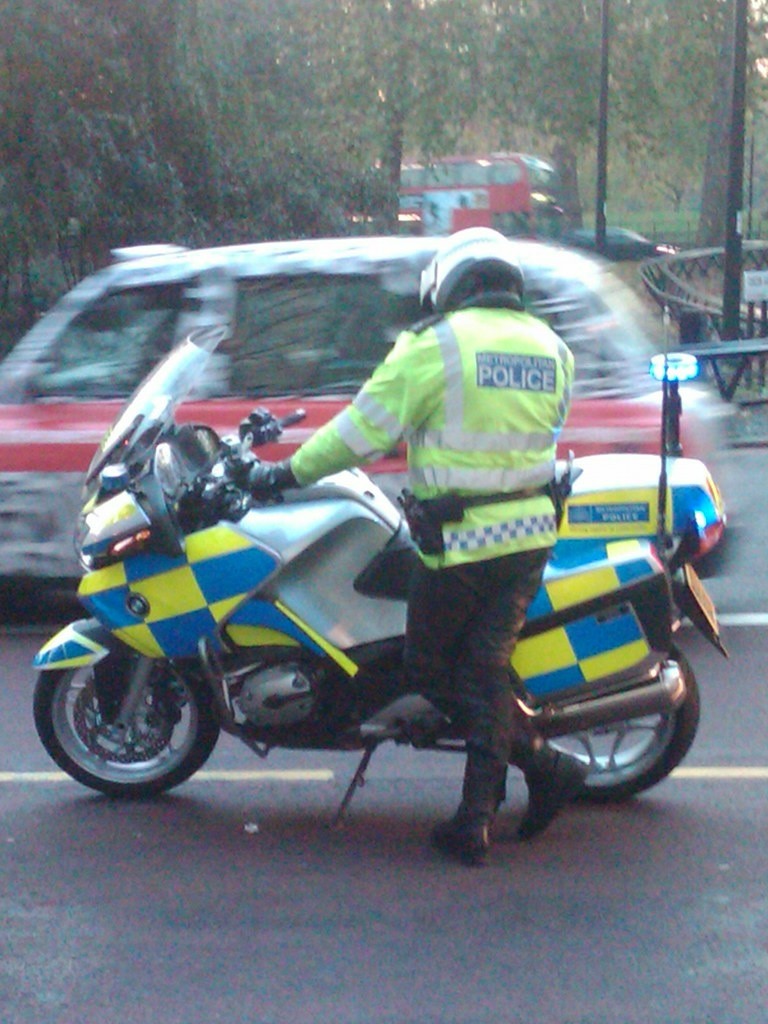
[360,154,562,237]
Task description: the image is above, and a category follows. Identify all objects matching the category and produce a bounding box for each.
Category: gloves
[237,457,299,501]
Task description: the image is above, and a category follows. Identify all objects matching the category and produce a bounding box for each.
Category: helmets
[419,226,524,312]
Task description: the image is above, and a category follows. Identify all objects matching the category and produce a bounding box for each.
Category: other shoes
[517,733,588,840]
[432,813,493,856]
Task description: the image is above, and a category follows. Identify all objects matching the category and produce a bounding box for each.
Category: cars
[547,226,680,259]
[0,237,741,633]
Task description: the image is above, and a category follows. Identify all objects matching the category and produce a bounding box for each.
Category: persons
[249,227,590,866]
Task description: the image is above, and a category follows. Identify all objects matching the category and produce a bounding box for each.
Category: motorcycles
[26,350,731,832]
